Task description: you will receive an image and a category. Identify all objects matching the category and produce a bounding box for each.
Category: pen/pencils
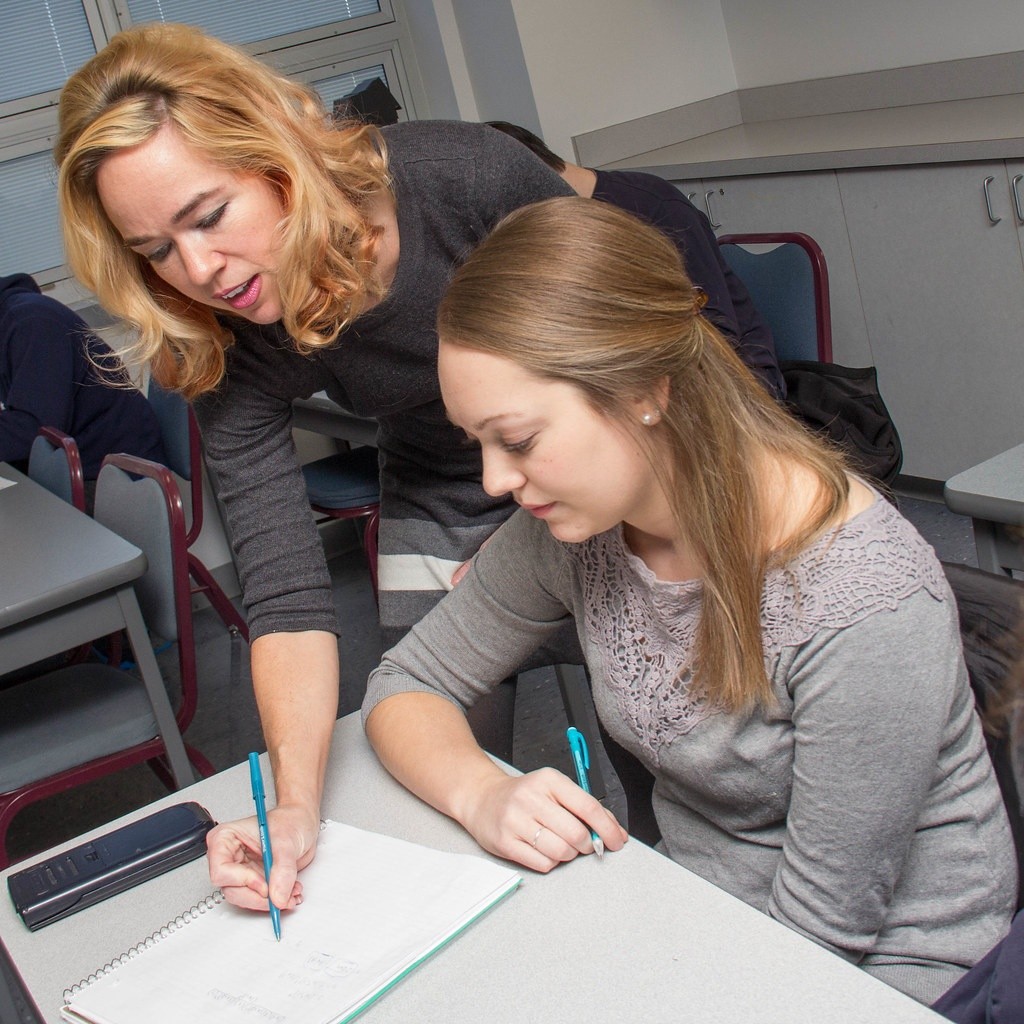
[249,750,281,941]
[566,727,604,858]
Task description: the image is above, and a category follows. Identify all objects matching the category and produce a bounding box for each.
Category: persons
[52,19,665,915]
[476,119,788,412]
[357,196,1024,1024]
[0,272,171,493]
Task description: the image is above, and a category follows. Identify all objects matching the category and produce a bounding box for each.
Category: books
[56,819,525,1022]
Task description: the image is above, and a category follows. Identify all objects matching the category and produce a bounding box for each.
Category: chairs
[0,425,94,693]
[299,445,381,617]
[939,562,1024,921]
[718,232,834,369]
[147,358,250,646]
[0,452,218,874]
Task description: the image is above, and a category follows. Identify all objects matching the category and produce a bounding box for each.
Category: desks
[0,710,955,1024]
[942,442,1024,583]
[0,458,195,792]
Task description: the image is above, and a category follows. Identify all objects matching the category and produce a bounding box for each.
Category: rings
[533,827,545,849]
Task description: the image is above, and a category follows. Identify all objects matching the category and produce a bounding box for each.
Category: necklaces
[340,204,371,315]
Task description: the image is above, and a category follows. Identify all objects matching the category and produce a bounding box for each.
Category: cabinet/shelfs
[668,171,875,369]
[837,158,1024,482]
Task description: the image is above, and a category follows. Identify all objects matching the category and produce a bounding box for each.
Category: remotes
[8,801,220,932]
